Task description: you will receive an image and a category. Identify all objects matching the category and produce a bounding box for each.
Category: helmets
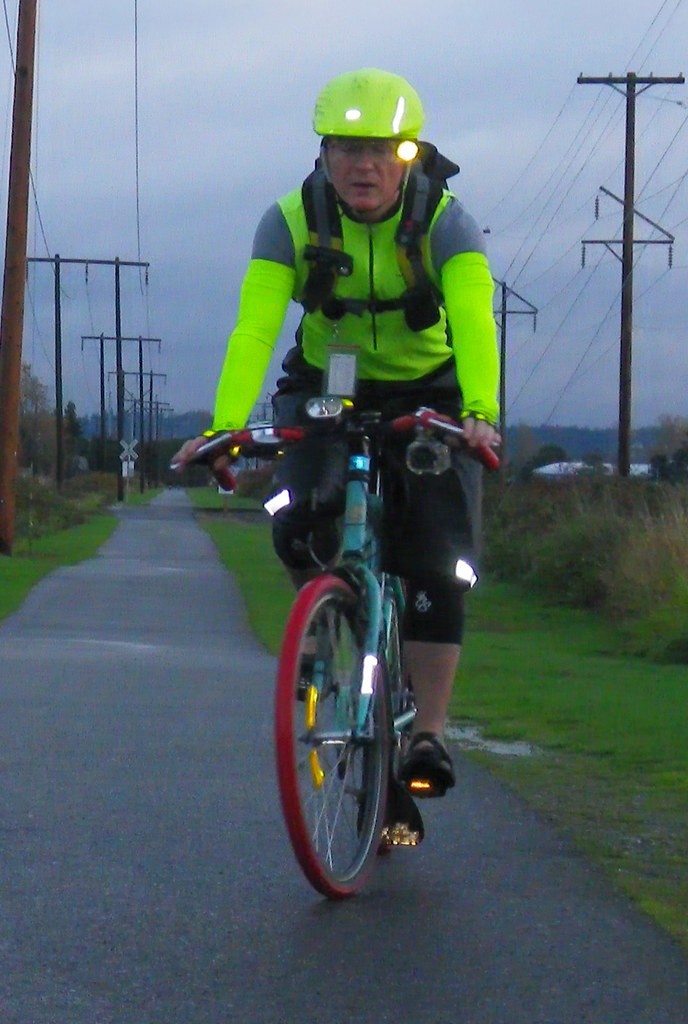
[311,67,425,139]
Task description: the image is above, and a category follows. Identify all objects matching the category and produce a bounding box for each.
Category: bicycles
[171,407,504,899]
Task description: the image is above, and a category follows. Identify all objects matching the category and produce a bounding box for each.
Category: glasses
[323,133,410,164]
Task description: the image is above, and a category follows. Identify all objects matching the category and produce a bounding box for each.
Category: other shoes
[398,732,455,799]
[297,655,334,702]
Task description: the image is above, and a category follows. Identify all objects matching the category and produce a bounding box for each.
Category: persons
[172,68,504,798]
